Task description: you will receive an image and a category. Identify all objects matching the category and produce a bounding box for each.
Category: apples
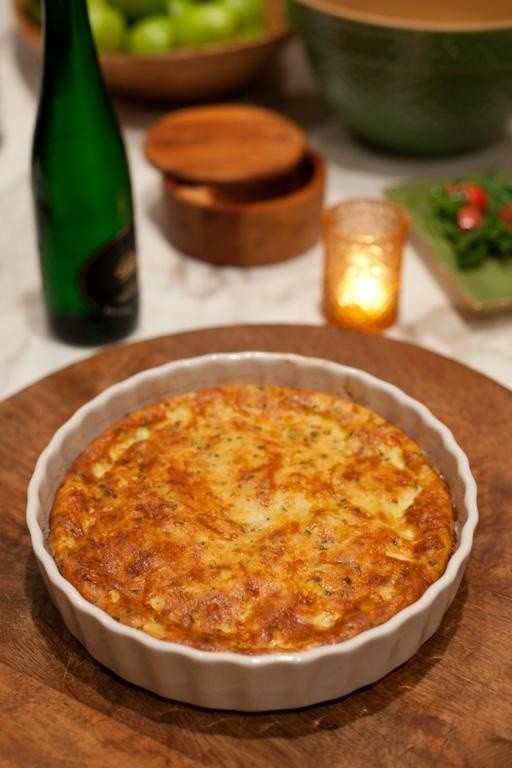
[88,0,265,53]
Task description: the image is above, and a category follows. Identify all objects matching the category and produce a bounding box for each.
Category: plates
[381,169,510,322]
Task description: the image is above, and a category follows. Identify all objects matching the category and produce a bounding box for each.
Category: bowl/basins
[152,149,325,266]
[293,0,512,159]
[25,346,482,717]
[11,0,288,102]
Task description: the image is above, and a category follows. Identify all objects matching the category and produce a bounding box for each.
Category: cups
[321,198,408,334]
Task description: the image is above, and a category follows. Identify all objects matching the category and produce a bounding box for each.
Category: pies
[50,383,454,660]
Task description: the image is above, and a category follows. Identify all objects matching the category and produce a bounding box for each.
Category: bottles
[31,1,142,340]
